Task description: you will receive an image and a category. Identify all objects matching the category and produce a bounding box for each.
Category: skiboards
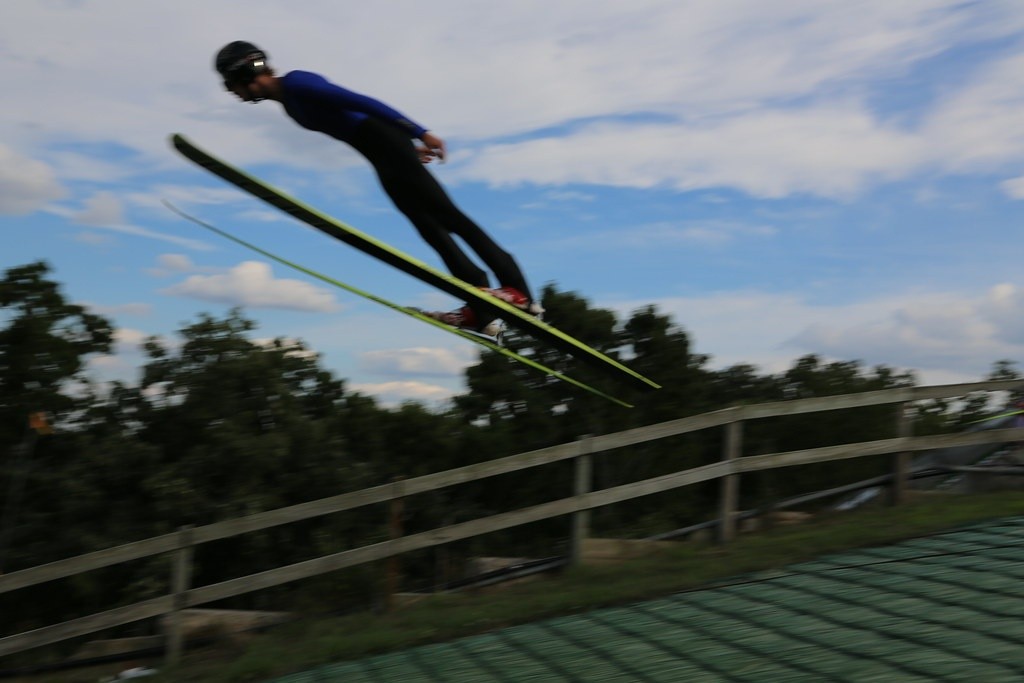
[156,129,665,410]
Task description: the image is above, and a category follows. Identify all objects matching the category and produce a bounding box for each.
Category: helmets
[216,40,267,90]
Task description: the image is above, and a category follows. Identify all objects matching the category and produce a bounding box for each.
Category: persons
[214,39,546,338]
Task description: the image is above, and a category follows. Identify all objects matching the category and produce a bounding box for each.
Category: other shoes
[423,304,507,342]
[480,285,540,317]
